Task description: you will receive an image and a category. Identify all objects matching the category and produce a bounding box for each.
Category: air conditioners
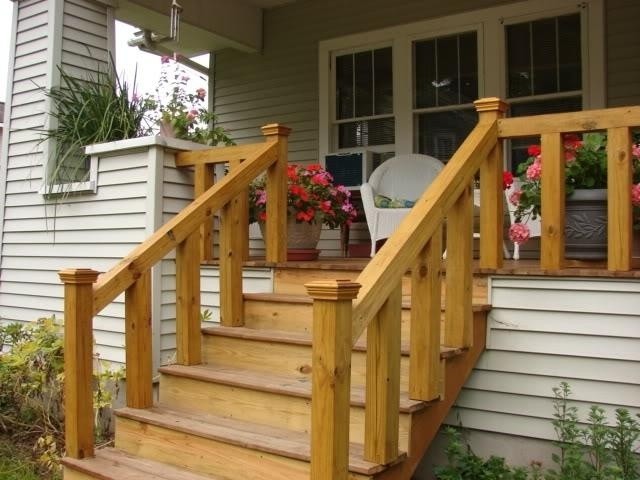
[322,152,374,190]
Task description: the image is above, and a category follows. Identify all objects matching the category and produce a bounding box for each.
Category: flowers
[503,133,640,244]
[130,57,238,147]
[248,164,357,228]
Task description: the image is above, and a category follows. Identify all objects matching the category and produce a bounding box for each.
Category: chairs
[505,182,541,259]
[360,154,445,258]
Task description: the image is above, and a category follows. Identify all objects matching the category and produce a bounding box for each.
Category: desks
[443,189,510,260]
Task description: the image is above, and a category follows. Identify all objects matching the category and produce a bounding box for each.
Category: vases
[160,117,188,139]
[257,216,322,249]
[565,189,608,259]
